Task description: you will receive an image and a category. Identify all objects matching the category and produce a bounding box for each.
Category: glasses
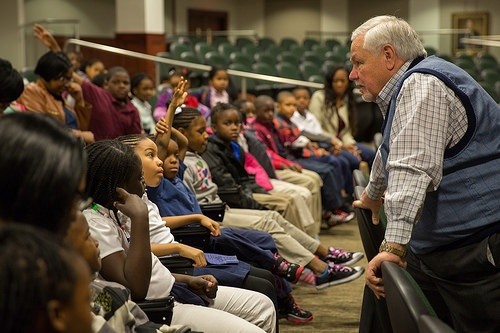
[59,76,75,84]
[76,187,93,212]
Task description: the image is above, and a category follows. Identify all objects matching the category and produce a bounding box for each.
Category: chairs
[353,161,455,332]
[157,35,500,104]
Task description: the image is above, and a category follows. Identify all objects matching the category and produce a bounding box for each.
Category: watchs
[378,238,408,258]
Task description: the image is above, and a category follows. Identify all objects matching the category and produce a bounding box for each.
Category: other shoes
[333,208,354,222]
[320,211,344,231]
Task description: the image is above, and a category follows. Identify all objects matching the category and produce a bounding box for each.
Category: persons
[116,135,279,333]
[348,14,500,333]
[75,139,276,333]
[0,26,385,333]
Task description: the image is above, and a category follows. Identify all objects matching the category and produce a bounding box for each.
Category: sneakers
[271,254,304,284]
[284,294,313,324]
[320,246,364,267]
[313,262,365,290]
